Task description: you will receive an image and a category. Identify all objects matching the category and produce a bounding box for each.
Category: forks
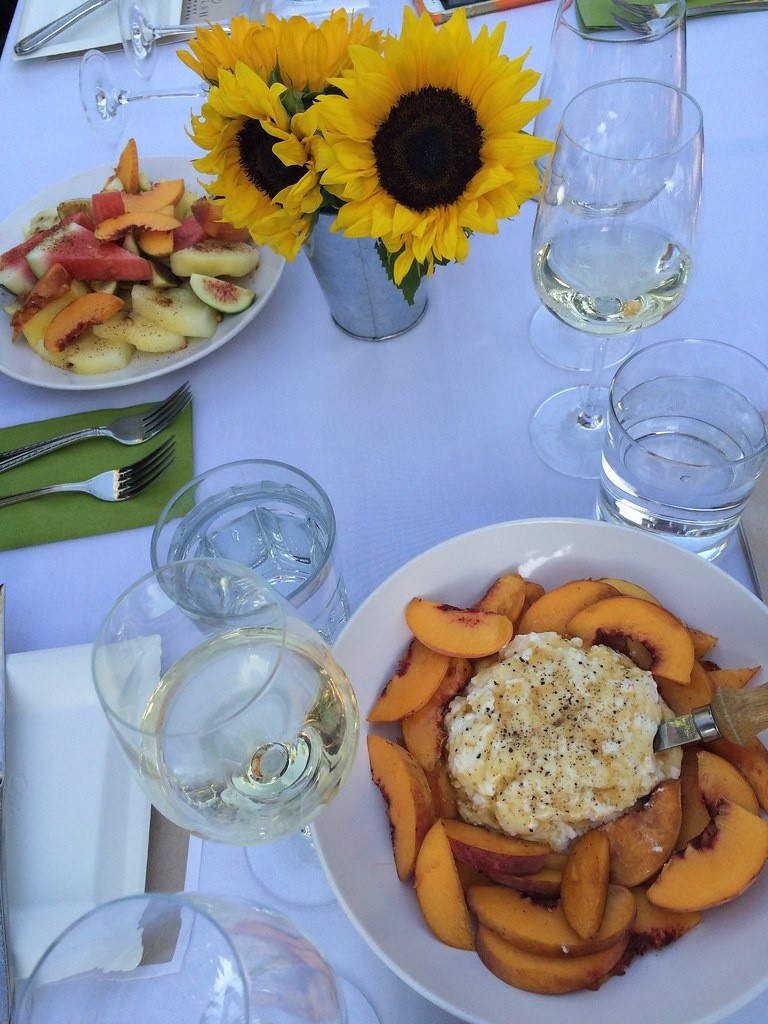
[0,434,177,511]
[0,381,192,475]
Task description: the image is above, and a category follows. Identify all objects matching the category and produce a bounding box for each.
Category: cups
[148,459,351,654]
[592,337,768,565]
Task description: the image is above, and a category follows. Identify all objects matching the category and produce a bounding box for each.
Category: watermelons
[0,189,210,298]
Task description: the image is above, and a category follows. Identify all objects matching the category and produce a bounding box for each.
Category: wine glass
[530,0,689,372]
[75,46,211,148]
[15,891,381,1024]
[116,0,395,79]
[529,75,705,483]
[88,556,362,910]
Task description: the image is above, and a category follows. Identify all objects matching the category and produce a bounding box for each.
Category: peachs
[366,574,768,997]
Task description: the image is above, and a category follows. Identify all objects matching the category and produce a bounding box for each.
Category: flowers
[180,6,565,308]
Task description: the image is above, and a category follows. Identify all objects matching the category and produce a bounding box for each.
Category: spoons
[14,0,111,56]
[650,680,767,751]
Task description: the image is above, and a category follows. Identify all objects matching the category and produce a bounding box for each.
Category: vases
[296,209,429,342]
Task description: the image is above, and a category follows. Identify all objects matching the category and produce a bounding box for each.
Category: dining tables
[2,1,766,1019]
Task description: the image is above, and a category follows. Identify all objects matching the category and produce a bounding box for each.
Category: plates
[302,513,766,1024]
[303,512,768,1024]
[10,0,184,62]
[0,155,287,390]
